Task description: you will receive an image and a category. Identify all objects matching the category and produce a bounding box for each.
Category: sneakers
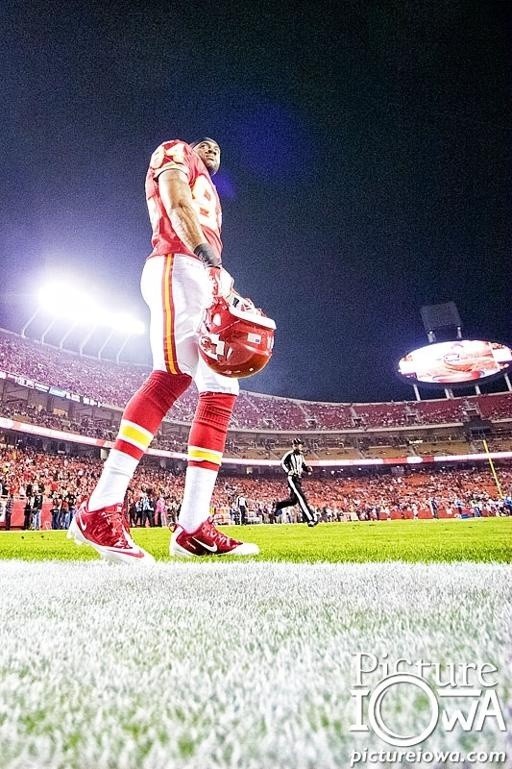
[307,519,319,527]
[168,516,260,558]
[273,501,278,516]
[67,497,155,564]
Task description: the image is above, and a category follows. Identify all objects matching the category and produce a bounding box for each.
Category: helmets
[197,297,276,378]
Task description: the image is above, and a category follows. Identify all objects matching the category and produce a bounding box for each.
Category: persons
[0,330,512,531]
[70,137,258,560]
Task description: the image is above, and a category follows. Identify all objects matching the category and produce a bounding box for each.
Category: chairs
[0,333,511,529]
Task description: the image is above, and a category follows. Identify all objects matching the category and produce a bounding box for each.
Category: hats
[290,437,305,445]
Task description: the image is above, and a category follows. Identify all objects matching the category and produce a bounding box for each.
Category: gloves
[205,262,234,299]
